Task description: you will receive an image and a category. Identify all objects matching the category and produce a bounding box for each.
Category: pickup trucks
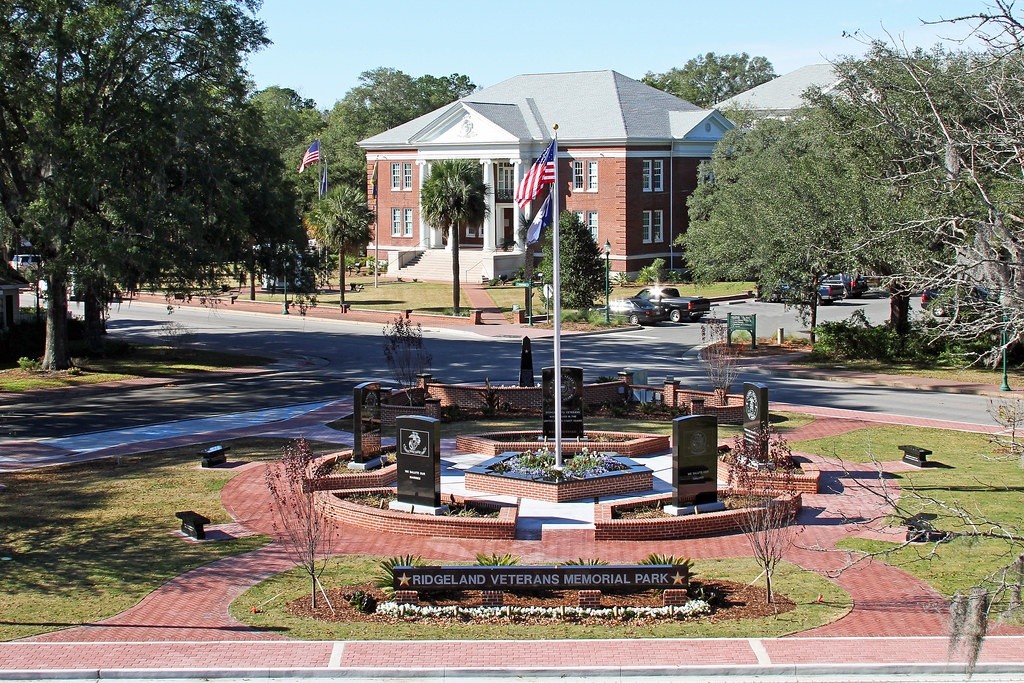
[757,280,844,306]
[628,287,710,322]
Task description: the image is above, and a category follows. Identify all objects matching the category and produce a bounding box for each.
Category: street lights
[1000,286,1011,391]
[278,242,291,315]
[603,239,612,324]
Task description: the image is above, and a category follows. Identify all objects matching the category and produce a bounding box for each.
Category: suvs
[10,255,44,272]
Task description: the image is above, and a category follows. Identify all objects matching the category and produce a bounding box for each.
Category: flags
[320,168,326,194]
[515,139,555,208]
[299,140,320,173]
[525,194,553,246]
[370,163,378,199]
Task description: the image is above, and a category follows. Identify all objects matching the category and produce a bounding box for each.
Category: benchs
[195,444,231,467]
[175,510,211,539]
[898,445,933,467]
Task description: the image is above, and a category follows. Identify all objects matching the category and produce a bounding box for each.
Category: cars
[820,271,869,299]
[921,285,991,317]
[589,298,666,324]
[29,272,77,299]
